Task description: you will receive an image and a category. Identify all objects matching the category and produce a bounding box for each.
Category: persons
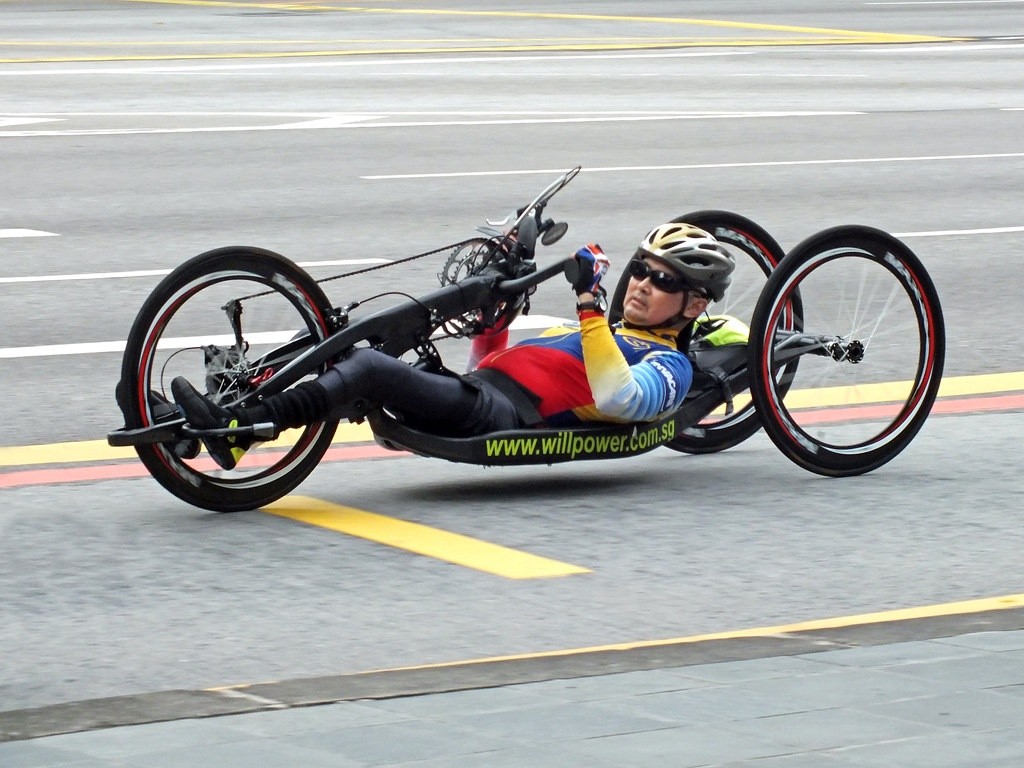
[114,223,737,474]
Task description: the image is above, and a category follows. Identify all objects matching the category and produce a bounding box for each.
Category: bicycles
[107,165,948,514]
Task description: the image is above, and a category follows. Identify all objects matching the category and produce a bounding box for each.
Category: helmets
[636,222,735,303]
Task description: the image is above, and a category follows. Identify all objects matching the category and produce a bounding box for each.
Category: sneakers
[147,390,202,460]
[171,375,254,470]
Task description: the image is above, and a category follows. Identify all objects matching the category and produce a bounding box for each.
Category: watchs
[576,298,606,312]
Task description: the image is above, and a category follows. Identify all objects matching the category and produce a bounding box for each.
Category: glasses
[629,258,709,299]
